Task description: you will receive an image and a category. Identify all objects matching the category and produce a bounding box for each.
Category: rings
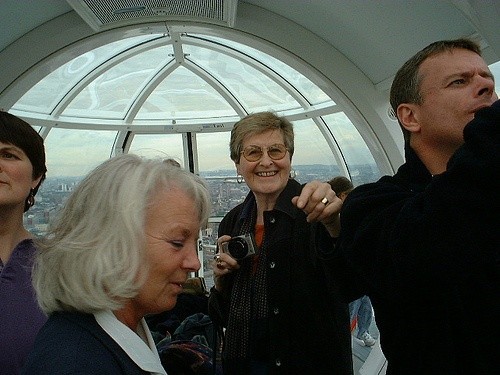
[320,196,330,205]
[216,261,226,269]
[214,253,221,261]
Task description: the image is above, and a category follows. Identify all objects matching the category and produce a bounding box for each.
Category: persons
[340,38,500,375]
[0,110,376,375]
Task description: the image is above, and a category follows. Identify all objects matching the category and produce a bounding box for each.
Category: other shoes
[357,333,376,347]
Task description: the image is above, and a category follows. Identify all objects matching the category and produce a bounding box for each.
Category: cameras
[221,233,259,260]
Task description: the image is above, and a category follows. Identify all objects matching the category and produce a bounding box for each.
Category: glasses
[238,144,291,162]
